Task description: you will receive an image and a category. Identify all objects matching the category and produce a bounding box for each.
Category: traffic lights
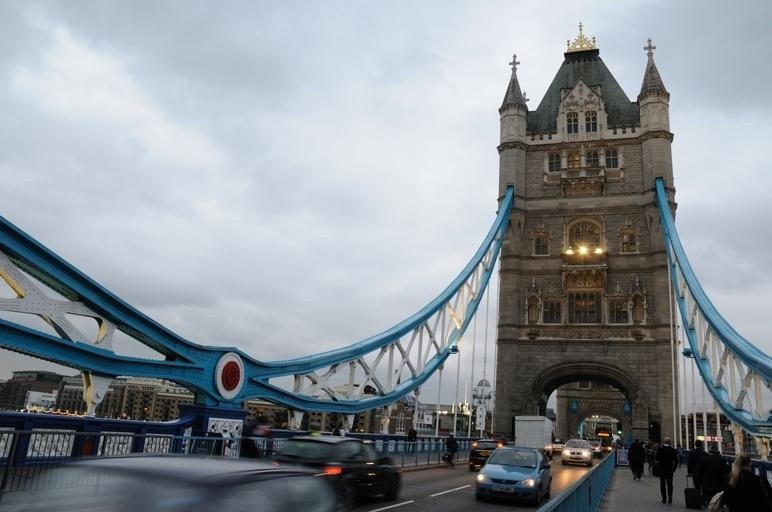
[618,431,622,435]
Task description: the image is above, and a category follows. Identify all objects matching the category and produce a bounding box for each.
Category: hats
[663,438,671,446]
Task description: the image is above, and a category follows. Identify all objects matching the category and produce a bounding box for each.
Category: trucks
[515,416,556,464]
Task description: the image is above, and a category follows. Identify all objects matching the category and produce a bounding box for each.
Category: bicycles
[444,447,458,469]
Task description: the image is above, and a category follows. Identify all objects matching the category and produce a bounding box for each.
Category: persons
[331,420,345,436]
[407,424,417,453]
[445,432,458,469]
[616,438,772,511]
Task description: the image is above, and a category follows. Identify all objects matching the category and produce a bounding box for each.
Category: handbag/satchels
[708,490,729,512]
[442,453,448,461]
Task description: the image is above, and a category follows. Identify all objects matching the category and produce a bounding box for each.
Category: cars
[270,434,400,509]
[553,432,620,467]
[469,439,508,472]
[475,446,552,507]
[0,452,337,512]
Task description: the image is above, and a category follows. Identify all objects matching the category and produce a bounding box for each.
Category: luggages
[685,475,699,508]
